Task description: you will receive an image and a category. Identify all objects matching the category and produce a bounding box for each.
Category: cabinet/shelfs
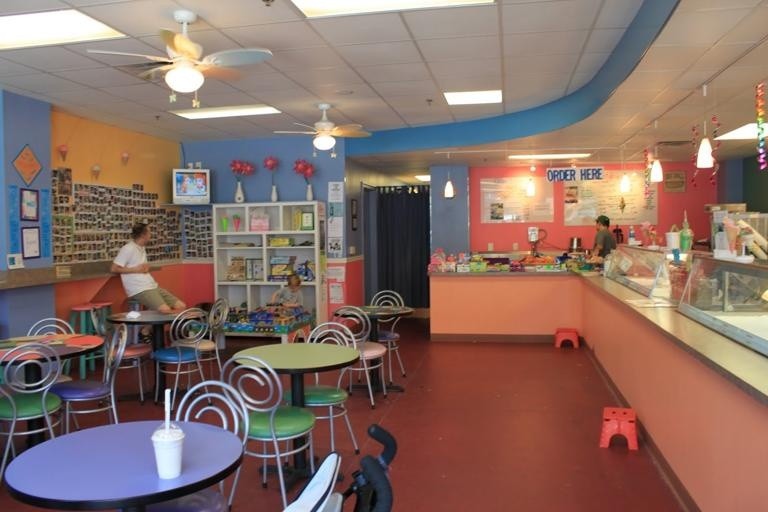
[209,200,326,339]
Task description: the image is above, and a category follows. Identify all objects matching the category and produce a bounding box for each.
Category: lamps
[695,84,714,168]
[166,66,205,92]
[313,136,334,151]
[648,117,664,183]
[442,151,455,199]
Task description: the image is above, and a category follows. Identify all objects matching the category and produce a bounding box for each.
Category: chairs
[327,306,387,408]
[144,381,249,512]
[219,356,316,512]
[0,287,229,476]
[363,290,407,386]
[282,321,360,465]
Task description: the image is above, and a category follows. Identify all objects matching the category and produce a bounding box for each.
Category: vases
[233,182,244,203]
[305,183,313,201]
[270,185,277,202]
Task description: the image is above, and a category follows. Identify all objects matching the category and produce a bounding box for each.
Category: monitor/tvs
[172,169,210,205]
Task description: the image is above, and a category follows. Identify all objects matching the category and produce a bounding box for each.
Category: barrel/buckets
[664,231,679,247]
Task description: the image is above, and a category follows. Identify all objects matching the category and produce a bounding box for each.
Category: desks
[4,421,243,512]
[233,343,361,492]
[333,306,411,398]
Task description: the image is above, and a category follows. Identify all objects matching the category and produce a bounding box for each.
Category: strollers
[283,424,398,512]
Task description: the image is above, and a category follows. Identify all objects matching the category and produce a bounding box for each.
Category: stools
[554,326,579,351]
[598,405,640,452]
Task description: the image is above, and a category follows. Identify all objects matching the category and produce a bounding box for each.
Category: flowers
[229,155,255,180]
[263,155,280,183]
[293,160,314,184]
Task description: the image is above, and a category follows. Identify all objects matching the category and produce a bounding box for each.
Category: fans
[272,103,371,139]
[87,8,273,82]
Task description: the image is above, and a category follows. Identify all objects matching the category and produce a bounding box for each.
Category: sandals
[138,332,153,345]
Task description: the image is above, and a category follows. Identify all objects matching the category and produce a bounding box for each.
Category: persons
[271,275,304,343]
[110,223,186,346]
[593,215,616,268]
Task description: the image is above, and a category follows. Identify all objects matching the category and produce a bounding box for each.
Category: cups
[150,421,186,479]
[187,163,193,169]
[680,230,691,252]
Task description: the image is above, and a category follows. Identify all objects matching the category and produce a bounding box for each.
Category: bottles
[196,161,201,169]
[613,223,621,240]
[628,226,635,244]
[715,225,727,249]
